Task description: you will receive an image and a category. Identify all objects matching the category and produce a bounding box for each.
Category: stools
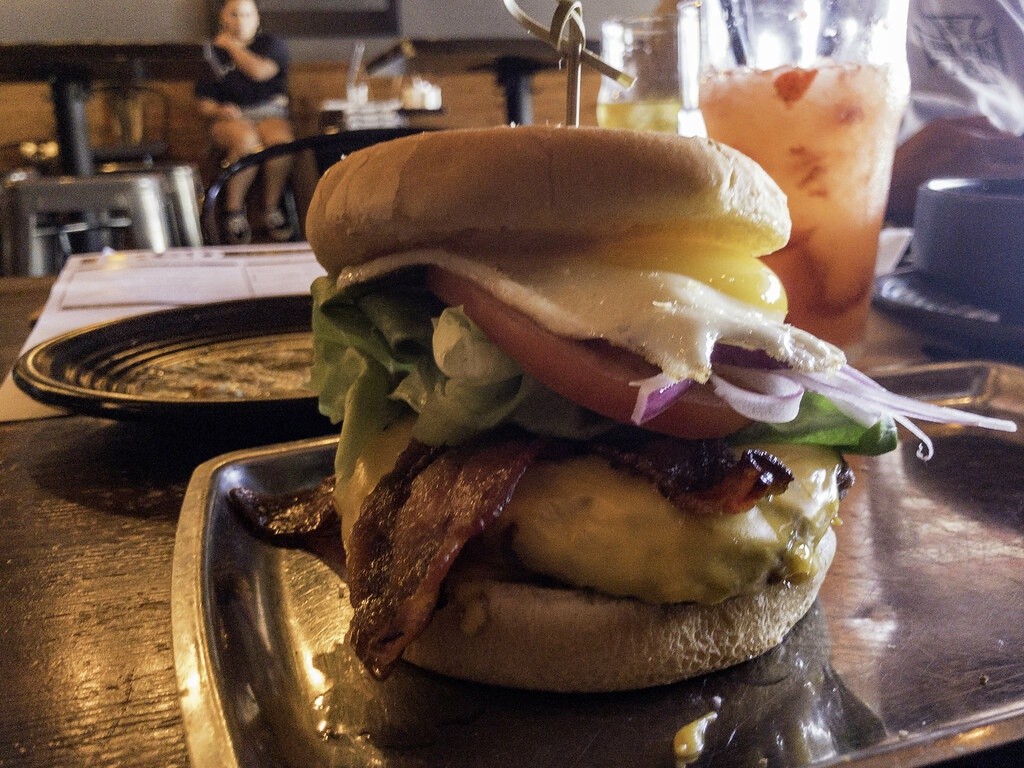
[17,180,175,279]
[123,161,199,246]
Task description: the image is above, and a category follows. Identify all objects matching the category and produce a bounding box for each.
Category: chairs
[202,126,465,245]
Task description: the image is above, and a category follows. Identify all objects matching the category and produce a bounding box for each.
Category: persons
[194,0,295,245]
[885,0,1024,230]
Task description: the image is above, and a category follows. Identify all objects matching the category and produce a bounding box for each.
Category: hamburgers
[228,125,1015,692]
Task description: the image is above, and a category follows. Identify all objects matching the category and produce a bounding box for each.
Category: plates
[871,269,1024,338]
[12,295,333,422]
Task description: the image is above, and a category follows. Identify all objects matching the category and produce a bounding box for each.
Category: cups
[909,178,1024,279]
[597,16,682,135]
[699,0,905,366]
[675,0,708,137]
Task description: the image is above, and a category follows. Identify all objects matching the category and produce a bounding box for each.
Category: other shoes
[224,202,252,246]
[262,211,292,242]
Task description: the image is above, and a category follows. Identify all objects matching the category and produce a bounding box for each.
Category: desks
[367,35,601,128]
[0,224,1024,768]
[1,41,237,247]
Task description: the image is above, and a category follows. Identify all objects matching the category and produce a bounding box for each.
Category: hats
[871,174,1024,346]
